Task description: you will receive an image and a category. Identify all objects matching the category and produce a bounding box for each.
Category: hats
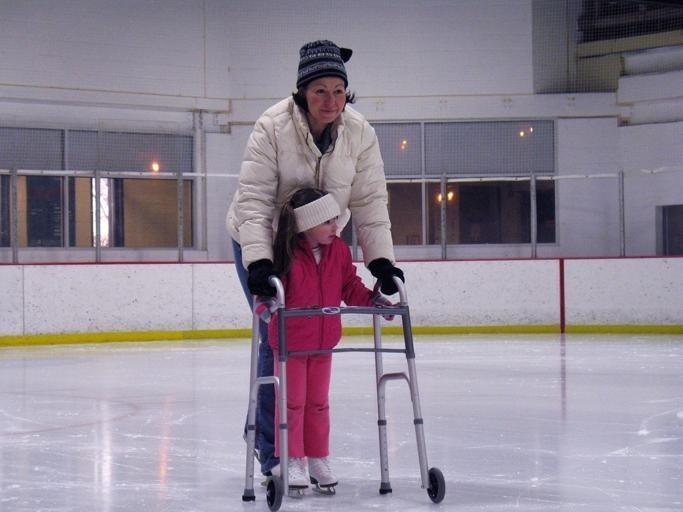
[296,39,352,88]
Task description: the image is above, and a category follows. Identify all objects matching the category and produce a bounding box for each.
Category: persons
[248,183,396,491]
[221,38,407,479]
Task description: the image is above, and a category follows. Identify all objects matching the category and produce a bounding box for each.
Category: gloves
[367,257,404,295]
[254,299,277,323]
[246,259,279,297]
[373,295,393,321]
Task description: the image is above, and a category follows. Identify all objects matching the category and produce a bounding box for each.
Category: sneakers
[308,456,337,487]
[287,456,310,489]
